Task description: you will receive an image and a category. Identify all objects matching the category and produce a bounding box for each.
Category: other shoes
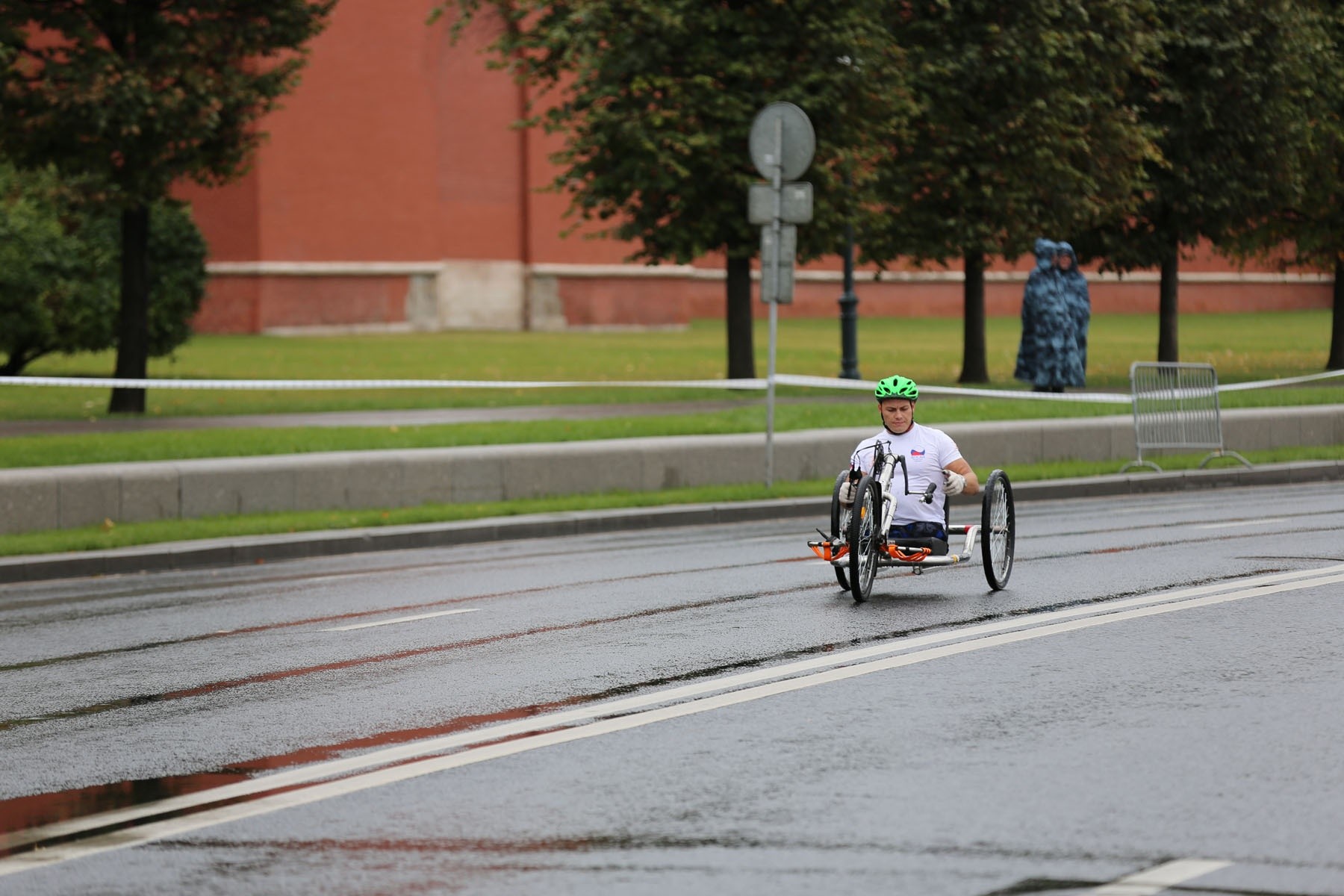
[1052,386,1065,392]
[1033,385,1049,392]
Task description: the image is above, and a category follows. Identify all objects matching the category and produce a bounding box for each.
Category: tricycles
[806,440,1018,602]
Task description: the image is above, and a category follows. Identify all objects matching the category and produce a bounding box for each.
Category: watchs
[960,474,968,490]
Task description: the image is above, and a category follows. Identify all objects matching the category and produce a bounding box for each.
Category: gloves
[941,469,964,496]
[839,482,857,503]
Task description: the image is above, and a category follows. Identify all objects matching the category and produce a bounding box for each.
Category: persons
[832,374,978,568]
[1014,238,1091,392]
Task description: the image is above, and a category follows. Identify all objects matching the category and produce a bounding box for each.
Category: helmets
[874,375,919,400]
